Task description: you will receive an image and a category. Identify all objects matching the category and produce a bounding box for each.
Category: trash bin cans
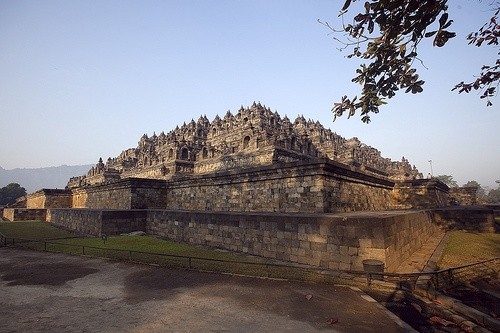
[362,259,385,280]
